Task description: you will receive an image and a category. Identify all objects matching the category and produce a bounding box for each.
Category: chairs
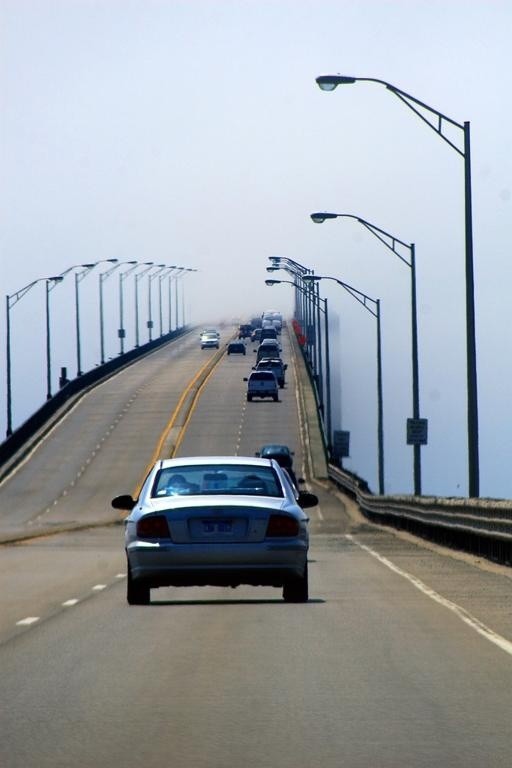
[238,475,267,496]
[169,475,186,485]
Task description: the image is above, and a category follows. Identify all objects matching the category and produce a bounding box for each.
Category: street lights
[3,273,64,438]
[43,261,95,401]
[71,257,200,376]
[301,272,386,498]
[307,209,423,495]
[263,253,334,458]
[314,72,483,496]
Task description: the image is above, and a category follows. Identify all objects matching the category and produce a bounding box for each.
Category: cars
[198,331,221,350]
[107,453,320,607]
[227,308,289,402]
[203,327,221,336]
[254,442,296,468]
[202,465,303,507]
